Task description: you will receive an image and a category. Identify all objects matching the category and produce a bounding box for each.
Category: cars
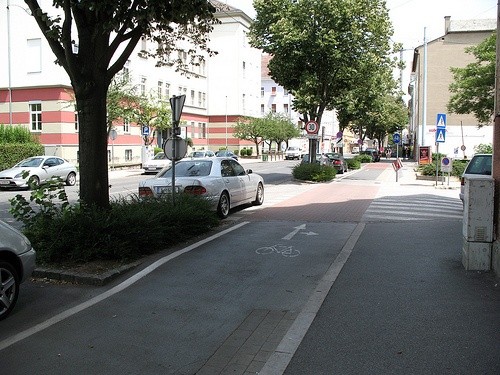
[299,152,349,173]
[460,153,494,203]
[188,150,217,157]
[137,156,266,217]
[216,151,238,162]
[0,220,36,321]
[139,152,175,174]
[0,155,77,190]
[341,153,358,167]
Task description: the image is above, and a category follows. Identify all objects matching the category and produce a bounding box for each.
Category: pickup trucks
[285,146,310,161]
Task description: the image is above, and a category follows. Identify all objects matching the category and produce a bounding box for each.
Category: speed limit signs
[305,120,319,134]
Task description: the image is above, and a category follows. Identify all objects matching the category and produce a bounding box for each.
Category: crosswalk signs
[436,129,445,143]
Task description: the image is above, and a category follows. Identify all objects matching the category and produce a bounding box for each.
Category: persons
[385,146,393,159]
[403,143,414,159]
